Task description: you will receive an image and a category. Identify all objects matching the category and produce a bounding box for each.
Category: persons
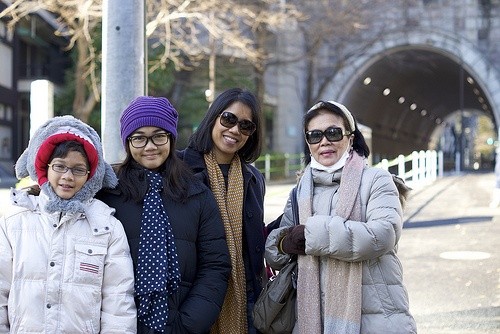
[91,96,232,334]
[176,88,283,334]
[265,100,417,334]
[0,115,138,334]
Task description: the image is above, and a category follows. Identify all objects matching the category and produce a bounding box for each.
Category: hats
[15,114,119,217]
[120,96,178,152]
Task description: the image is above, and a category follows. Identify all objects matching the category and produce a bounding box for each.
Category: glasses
[47,164,90,176]
[216,111,257,137]
[127,132,171,149]
[304,127,350,144]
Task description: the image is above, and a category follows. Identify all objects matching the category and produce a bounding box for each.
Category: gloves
[282,224,306,256]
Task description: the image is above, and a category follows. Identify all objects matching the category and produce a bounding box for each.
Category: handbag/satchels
[252,259,299,334]
